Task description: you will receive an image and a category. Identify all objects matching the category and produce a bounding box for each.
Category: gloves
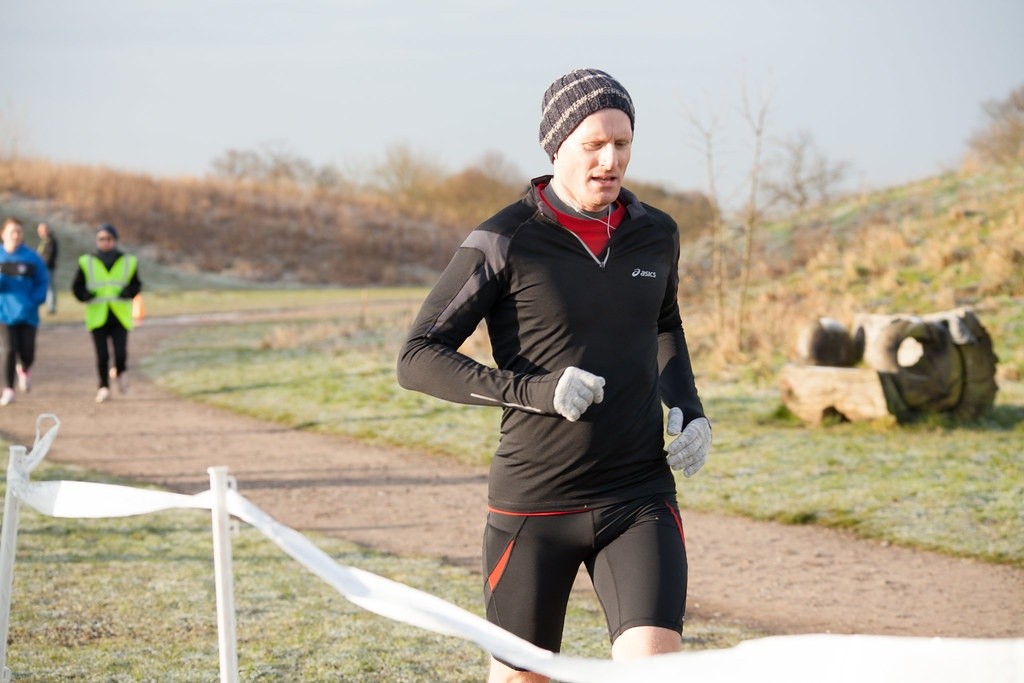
[663,406,711,479]
[553,368,603,422]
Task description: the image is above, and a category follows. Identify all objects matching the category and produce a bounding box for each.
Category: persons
[0,217,50,403]
[398,69,715,682]
[72,224,143,402]
[36,222,58,314]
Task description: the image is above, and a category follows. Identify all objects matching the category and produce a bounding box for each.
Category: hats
[539,69,639,164]
[95,223,117,241]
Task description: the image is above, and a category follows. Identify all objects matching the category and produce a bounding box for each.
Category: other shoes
[98,385,109,404]
[1,386,15,406]
[16,363,32,392]
[117,371,129,393]
